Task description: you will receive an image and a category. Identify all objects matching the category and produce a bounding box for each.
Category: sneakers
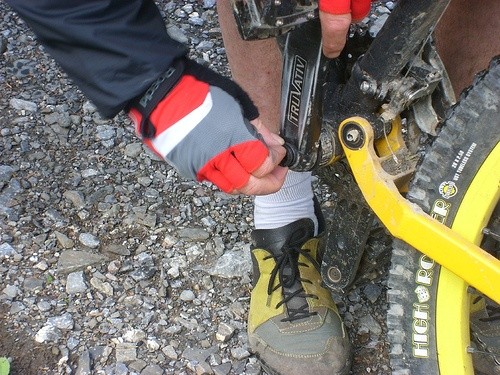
[246,190,352,375]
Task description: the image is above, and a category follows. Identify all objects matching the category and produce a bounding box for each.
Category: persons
[0,0,500,375]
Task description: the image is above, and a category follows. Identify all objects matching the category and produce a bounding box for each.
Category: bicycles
[233,0,500,375]
